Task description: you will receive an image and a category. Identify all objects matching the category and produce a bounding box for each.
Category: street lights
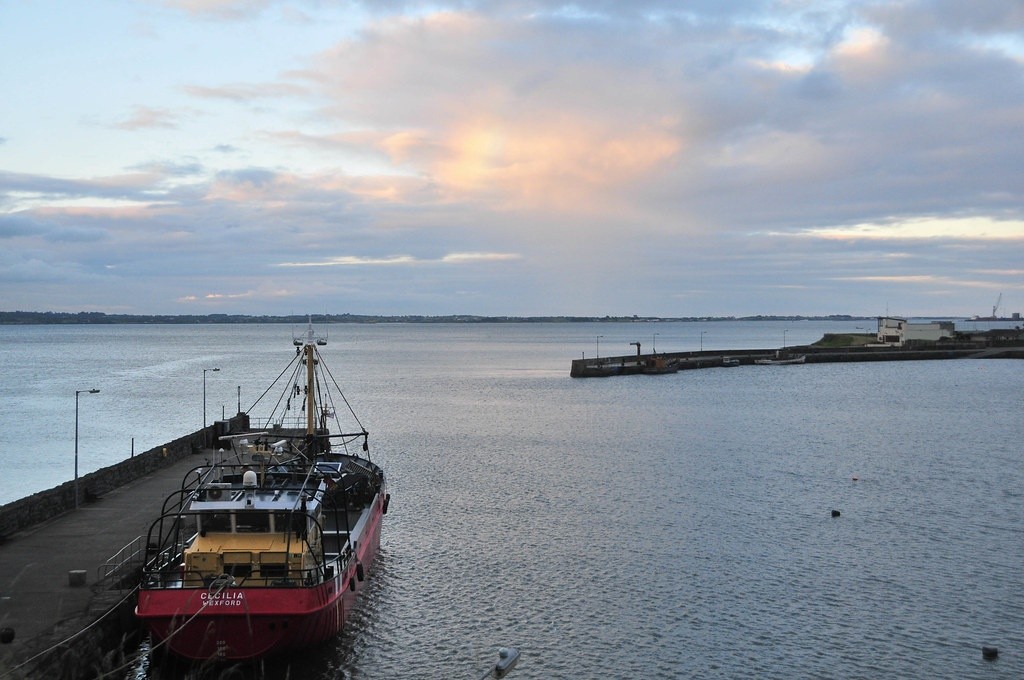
[75,389,100,509]
[597,335,603,364]
[866,329,871,347]
[654,331,660,355]
[204,367,220,448]
[784,329,788,349]
[701,330,707,352]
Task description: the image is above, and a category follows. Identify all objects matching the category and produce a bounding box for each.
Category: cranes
[992,293,1002,315]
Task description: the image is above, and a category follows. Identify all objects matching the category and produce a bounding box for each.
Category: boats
[642,357,679,374]
[754,350,806,365]
[721,357,739,367]
[134,317,390,663]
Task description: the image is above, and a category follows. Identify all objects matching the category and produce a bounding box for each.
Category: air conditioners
[207,482,232,501]
